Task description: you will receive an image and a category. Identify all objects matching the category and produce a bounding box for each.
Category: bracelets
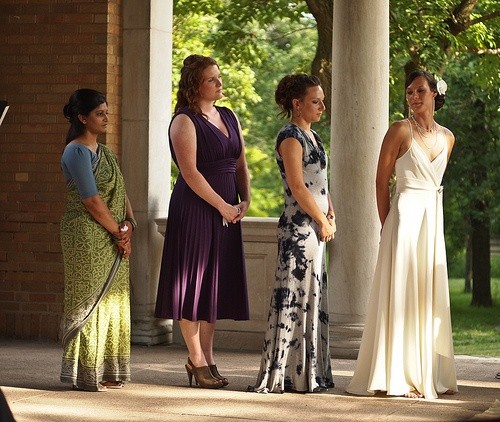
[125,217,137,231]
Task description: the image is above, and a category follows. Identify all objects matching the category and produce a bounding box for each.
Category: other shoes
[98,379,126,392]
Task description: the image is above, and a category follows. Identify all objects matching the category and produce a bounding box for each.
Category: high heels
[184,357,223,388]
[211,364,229,386]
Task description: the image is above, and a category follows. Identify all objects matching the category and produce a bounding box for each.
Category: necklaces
[411,114,439,148]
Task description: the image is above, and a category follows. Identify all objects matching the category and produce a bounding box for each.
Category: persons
[345,71,458,398]
[154,55,251,388]
[59,88,138,392]
[247,75,336,394]
